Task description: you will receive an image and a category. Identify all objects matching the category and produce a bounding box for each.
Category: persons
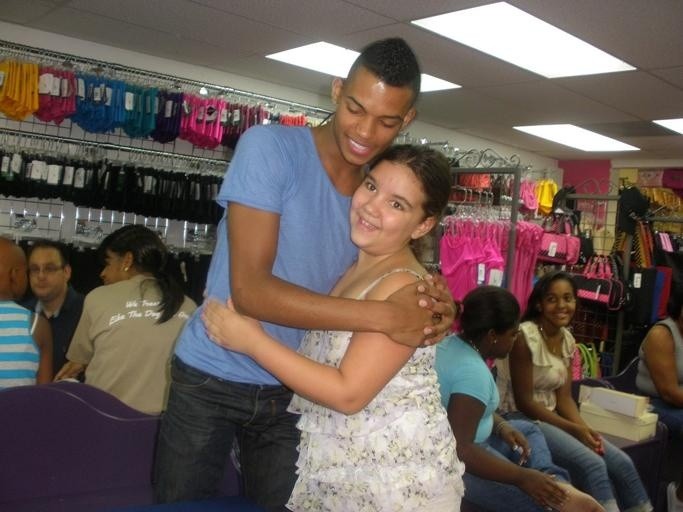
[437,270,652,511]
[152,37,466,511]
[1,224,197,416]
[636,284,683,487]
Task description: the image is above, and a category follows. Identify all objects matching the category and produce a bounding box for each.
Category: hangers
[1,126,231,179]
[1,42,309,123]
[439,184,524,229]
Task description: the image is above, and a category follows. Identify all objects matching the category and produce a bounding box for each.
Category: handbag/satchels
[537,212,673,328]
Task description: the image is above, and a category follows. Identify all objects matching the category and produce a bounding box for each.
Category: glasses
[28,263,64,274]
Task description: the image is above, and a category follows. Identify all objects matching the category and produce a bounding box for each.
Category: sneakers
[667,481,683,512]
